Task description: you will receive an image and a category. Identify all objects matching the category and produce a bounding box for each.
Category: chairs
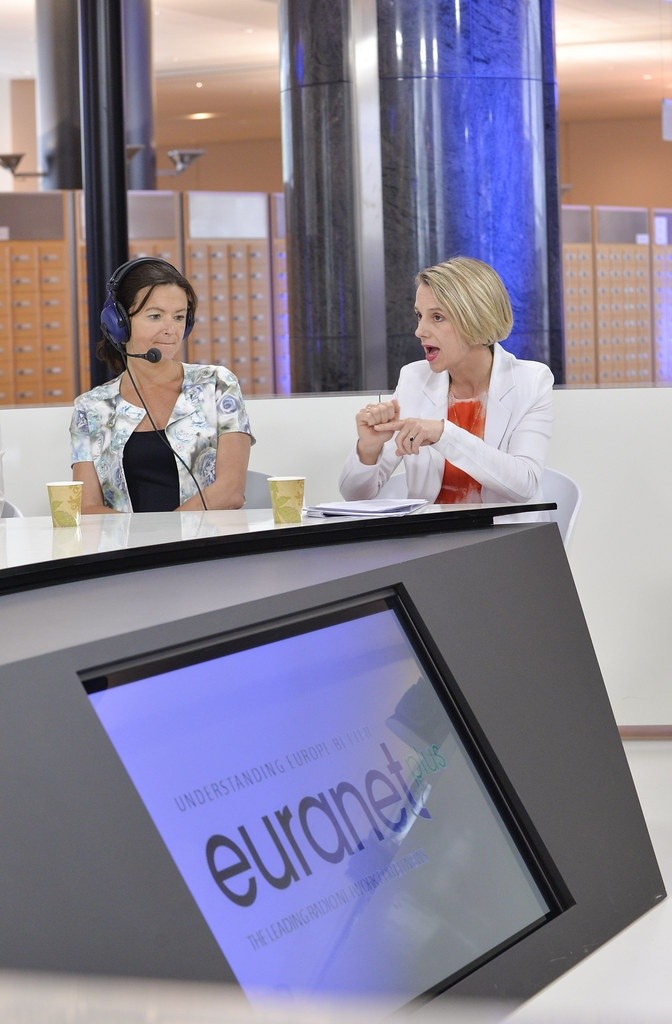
[364,463,582,550]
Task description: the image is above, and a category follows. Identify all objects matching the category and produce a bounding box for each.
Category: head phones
[100,257,195,343]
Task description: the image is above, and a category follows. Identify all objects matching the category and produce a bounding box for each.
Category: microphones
[100,324,162,363]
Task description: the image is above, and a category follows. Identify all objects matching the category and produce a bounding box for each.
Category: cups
[267,475,306,524]
[46,480,84,527]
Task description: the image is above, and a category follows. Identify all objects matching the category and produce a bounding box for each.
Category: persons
[337,255,559,524]
[70,257,257,513]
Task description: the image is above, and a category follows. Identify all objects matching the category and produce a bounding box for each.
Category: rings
[406,435,416,442]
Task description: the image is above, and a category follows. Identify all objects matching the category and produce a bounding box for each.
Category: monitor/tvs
[77,593,563,1024]
